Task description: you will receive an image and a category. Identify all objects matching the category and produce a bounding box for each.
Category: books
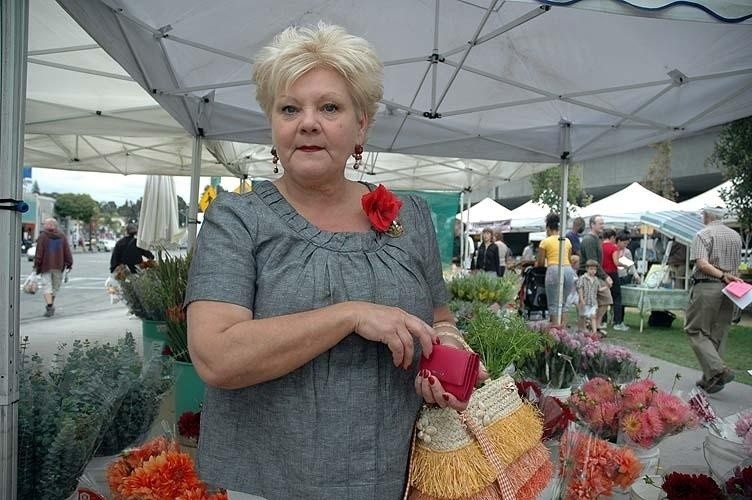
[722,275,752,310]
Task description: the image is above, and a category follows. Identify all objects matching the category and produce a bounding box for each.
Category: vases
[610,448,661,500]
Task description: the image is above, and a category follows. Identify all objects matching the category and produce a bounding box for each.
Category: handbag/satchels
[408,322,554,499]
[647,311,676,328]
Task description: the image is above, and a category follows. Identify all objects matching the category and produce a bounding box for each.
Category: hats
[699,198,727,216]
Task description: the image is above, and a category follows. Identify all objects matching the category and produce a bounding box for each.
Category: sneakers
[696,379,706,389]
[601,322,607,328]
[613,322,630,331]
[706,368,736,394]
[45,306,52,317]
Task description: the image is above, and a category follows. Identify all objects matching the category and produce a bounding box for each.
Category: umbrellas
[506,188,582,234]
[679,173,752,263]
[138,173,181,263]
[640,205,708,289]
[572,175,678,334]
[457,191,514,233]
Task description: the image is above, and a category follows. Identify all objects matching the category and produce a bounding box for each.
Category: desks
[617,284,689,333]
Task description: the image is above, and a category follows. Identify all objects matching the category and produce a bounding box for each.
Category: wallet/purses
[415,344,480,403]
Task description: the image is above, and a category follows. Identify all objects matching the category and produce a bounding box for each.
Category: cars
[26,243,36,262]
[72,238,130,252]
[176,232,189,251]
[20,241,30,253]
[739,250,751,266]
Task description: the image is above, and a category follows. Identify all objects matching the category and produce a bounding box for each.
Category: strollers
[515,265,548,320]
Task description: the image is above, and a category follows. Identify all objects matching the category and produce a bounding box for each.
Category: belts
[693,278,724,284]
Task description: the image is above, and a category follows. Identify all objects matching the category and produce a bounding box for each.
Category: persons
[182,16,489,499]
[109,221,156,279]
[685,203,744,394]
[32,217,74,317]
[435,200,676,340]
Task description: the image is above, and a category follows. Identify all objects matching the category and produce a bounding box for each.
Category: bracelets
[718,269,728,282]
[431,319,464,330]
[432,329,465,346]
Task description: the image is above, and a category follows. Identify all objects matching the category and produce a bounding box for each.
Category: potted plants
[104,246,207,440]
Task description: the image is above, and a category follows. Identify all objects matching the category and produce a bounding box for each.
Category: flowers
[106,440,232,500]
[578,378,695,444]
[359,182,404,232]
[443,267,646,439]
[550,428,642,499]
